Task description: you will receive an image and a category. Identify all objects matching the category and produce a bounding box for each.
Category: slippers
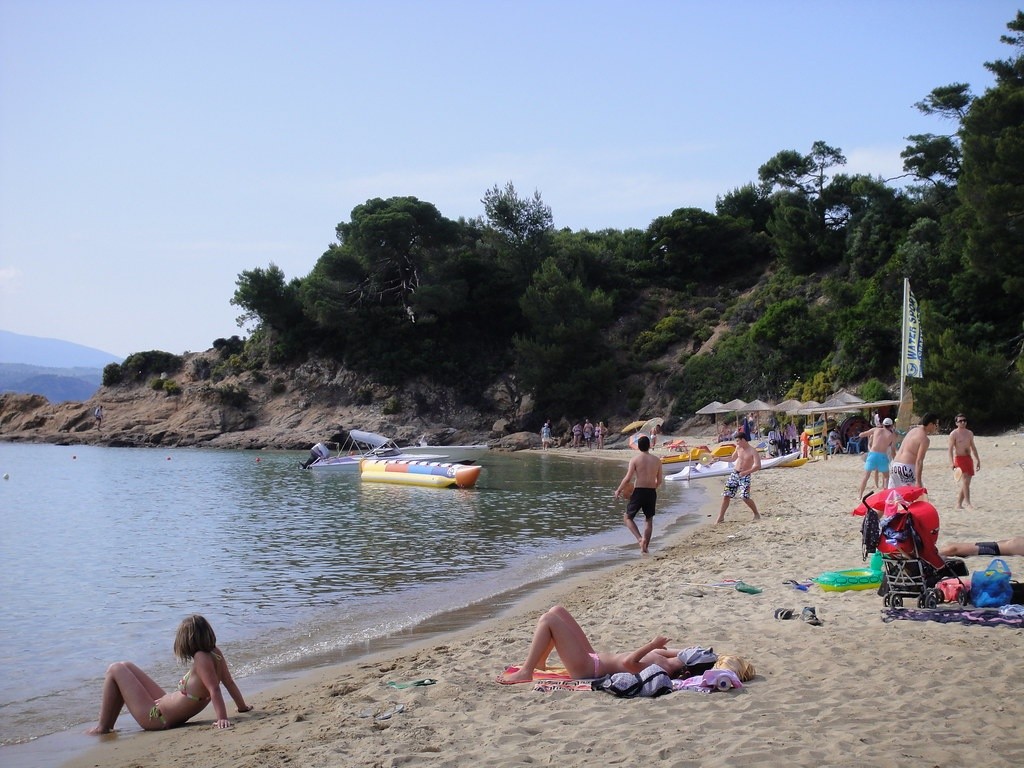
[359,705,380,718]
[378,704,403,720]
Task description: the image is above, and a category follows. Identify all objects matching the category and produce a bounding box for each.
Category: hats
[883,418,893,426]
[544,423,549,426]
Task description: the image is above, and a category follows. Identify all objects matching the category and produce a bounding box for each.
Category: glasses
[957,419,966,423]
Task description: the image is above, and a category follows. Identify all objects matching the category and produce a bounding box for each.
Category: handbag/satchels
[971,559,1013,608]
[1009,580,1024,605]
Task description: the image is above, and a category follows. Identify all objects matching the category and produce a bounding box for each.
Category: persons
[888,411,938,487]
[496,606,717,683]
[828,428,848,458]
[858,418,898,498]
[540,420,552,452]
[939,536,1024,556]
[715,432,761,525]
[81,615,253,735]
[650,424,660,451]
[572,417,608,451]
[94,405,103,432]
[717,422,732,443]
[948,413,980,510]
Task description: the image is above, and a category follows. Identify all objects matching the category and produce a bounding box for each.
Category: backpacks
[603,663,673,699]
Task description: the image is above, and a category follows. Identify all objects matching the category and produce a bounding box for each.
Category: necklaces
[615,436,662,553]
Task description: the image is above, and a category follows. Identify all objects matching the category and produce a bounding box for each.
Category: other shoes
[774,608,796,621]
[802,605,821,624]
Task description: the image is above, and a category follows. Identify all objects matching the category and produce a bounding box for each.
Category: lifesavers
[811,549,891,593]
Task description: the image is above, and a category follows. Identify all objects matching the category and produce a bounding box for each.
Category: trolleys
[862,491,969,609]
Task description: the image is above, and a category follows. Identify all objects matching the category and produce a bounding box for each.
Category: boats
[659,444,765,463]
[809,430,836,446]
[358,458,482,489]
[810,447,824,457]
[664,451,799,481]
[298,430,489,472]
[804,419,837,435]
[783,458,808,467]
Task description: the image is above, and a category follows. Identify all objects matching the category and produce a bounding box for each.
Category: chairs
[654,439,693,452]
[829,437,861,453]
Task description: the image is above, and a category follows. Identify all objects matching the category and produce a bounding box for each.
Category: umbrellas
[694,391,867,441]
[621,417,665,450]
